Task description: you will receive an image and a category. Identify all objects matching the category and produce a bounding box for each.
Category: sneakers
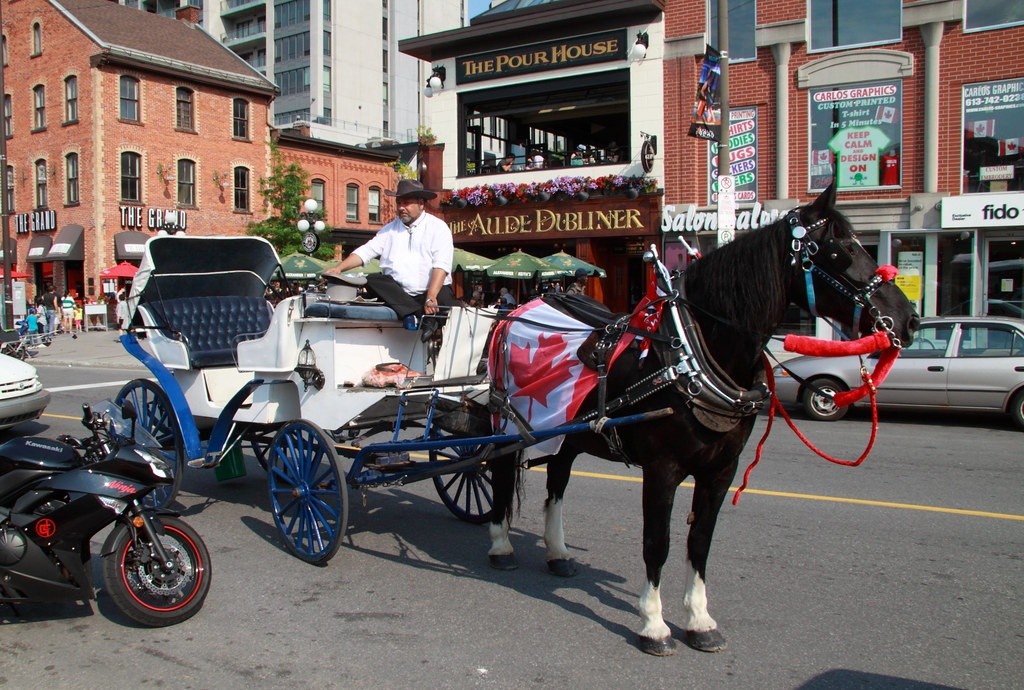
[421,318,438,343]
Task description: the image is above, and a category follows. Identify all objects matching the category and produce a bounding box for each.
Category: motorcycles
[0,397,214,629]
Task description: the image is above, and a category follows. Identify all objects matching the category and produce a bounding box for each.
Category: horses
[487,179,923,657]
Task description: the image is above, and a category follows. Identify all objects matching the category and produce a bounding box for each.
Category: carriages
[114,177,921,662]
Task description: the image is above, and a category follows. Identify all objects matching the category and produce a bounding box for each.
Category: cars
[773,315,1024,431]
[1,351,52,428]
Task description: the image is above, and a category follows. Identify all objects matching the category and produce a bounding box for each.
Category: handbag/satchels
[362,362,425,389]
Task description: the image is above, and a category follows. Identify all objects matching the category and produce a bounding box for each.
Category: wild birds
[72,334,78,339]
[43,341,52,346]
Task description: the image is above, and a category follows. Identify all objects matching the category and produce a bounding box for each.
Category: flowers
[443,172,656,206]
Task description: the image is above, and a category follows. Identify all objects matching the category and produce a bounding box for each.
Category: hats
[573,268,591,280]
[384,179,437,199]
[498,288,508,294]
[29,308,35,312]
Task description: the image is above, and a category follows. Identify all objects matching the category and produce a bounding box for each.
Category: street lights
[296,198,327,256]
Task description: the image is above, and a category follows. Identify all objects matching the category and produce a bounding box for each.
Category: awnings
[0,224,85,263]
[114,232,151,260]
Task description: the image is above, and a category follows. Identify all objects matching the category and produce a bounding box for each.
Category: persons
[326,178,463,331]
[496,142,622,171]
[468,267,591,310]
[25,285,127,347]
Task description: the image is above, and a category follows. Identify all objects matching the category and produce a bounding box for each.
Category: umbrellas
[274,247,608,305]
[102,262,139,279]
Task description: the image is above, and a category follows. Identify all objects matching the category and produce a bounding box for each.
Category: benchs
[139,294,275,370]
[294,302,421,327]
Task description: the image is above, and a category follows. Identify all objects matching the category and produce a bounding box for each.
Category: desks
[84,304,109,332]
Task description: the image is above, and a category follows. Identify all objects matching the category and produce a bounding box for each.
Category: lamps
[626,188,638,199]
[497,195,508,206]
[539,191,550,202]
[424,66,446,96]
[633,32,649,59]
[578,191,589,202]
[156,164,175,181]
[213,170,230,190]
[456,199,467,209]
